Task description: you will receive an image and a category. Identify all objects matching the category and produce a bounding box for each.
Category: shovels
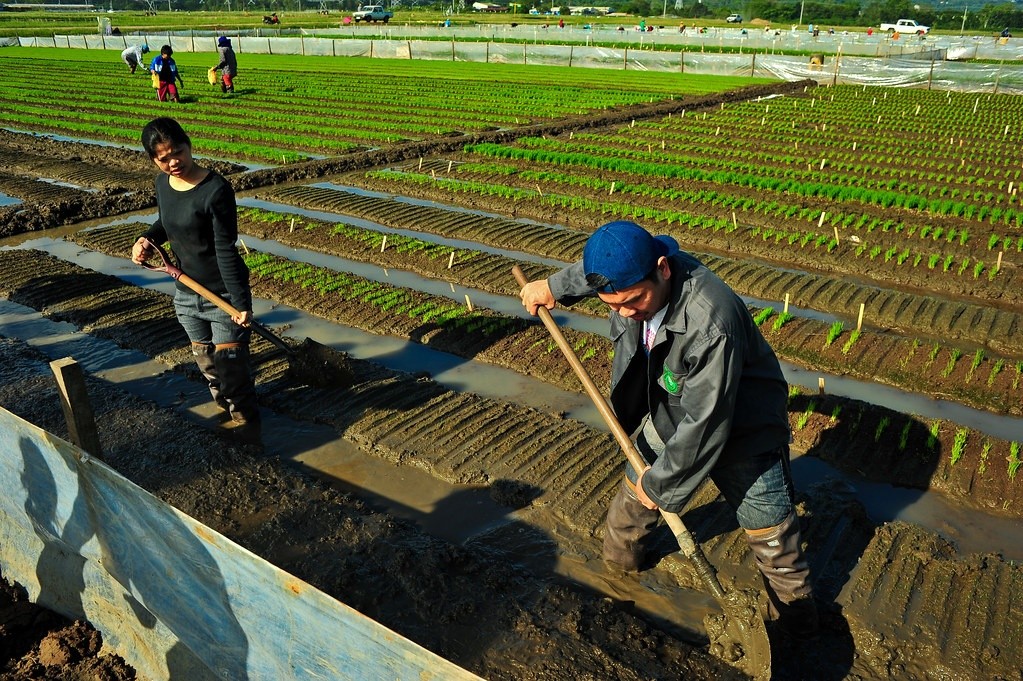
[507,263,775,681]
[138,239,359,398]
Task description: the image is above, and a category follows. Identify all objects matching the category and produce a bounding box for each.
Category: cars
[726,14,742,24]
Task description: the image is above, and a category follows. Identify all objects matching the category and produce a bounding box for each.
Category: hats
[141,45,150,53]
[218,36,230,46]
[584,220,680,293]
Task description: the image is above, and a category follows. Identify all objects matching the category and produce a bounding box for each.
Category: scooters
[261,13,280,25]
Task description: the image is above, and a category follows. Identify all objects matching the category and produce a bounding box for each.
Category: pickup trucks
[352,6,393,24]
[879,19,930,35]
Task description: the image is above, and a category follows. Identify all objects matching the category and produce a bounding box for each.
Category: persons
[637,20,1012,45]
[211,35,238,93]
[129,119,254,421]
[121,45,150,75]
[515,219,820,642]
[541,24,549,28]
[150,45,184,103]
[443,19,450,28]
[270,12,278,23]
[559,18,564,27]
